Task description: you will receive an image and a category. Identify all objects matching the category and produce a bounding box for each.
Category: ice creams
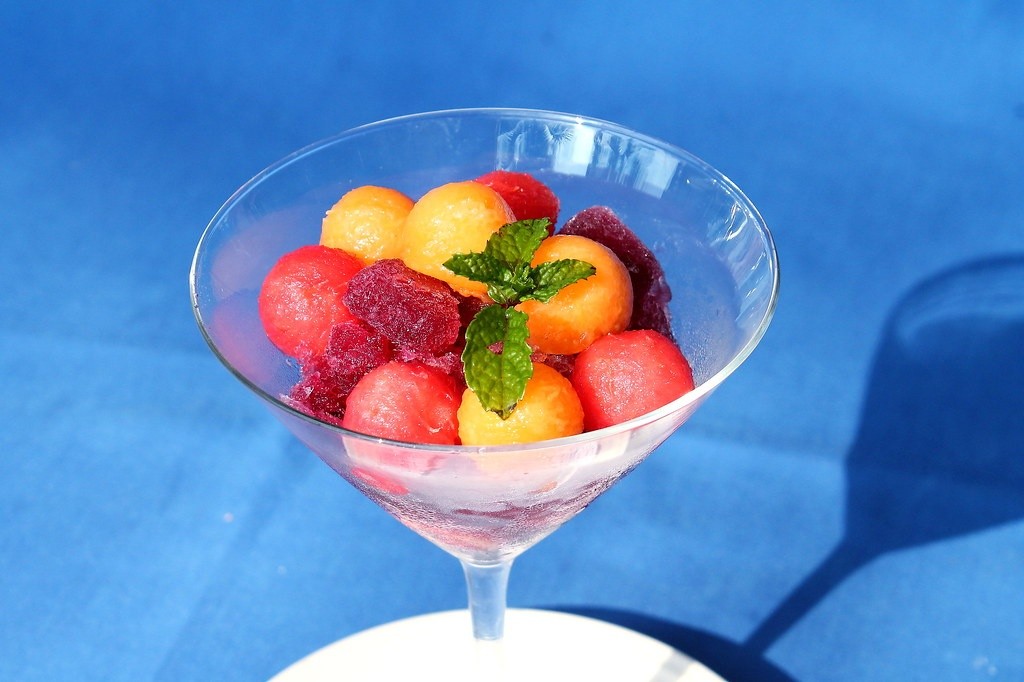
[254,167,701,554]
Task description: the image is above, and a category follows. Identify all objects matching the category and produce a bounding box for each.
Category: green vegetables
[442,214,596,422]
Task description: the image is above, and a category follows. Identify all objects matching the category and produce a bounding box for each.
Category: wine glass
[189,108,781,682]
